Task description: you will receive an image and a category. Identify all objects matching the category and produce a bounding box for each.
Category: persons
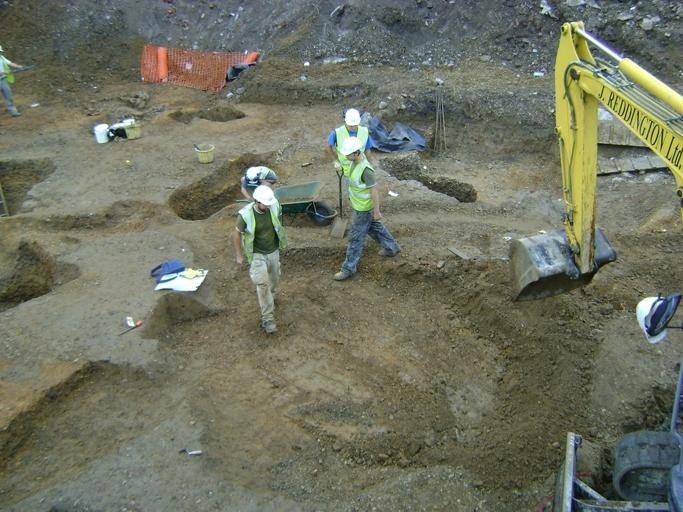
[331,137,401,280]
[233,185,284,335]
[239,166,276,200]
[327,109,373,199]
[0,45,29,118]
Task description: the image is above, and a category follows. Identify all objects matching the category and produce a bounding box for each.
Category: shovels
[330,165,349,239]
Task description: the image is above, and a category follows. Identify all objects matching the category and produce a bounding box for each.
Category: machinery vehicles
[541,15,682,512]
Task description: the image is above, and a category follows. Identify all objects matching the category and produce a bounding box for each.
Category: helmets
[244,167,261,188]
[253,185,277,206]
[635,296,666,344]
[344,109,360,126]
[341,136,362,155]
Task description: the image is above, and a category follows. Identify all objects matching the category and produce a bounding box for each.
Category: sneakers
[10,110,21,116]
[377,245,402,256]
[263,319,278,334]
[334,270,352,281]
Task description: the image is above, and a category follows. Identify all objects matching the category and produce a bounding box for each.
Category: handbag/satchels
[150,259,184,284]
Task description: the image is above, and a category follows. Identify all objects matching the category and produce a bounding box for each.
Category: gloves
[333,159,341,171]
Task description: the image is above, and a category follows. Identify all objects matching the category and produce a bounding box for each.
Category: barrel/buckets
[194,143,214,162]
[125,124,142,140]
[94,124,109,144]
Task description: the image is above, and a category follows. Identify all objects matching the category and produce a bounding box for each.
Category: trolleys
[230,177,325,229]
